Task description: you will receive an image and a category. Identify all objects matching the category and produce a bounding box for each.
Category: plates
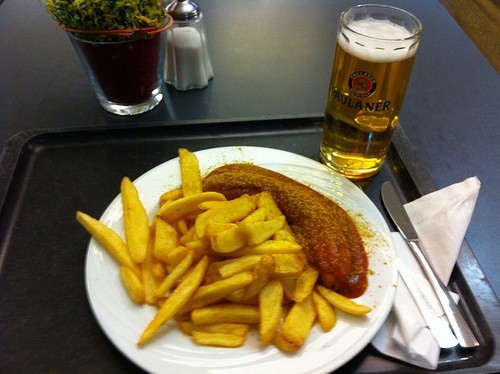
[85,146,397,374]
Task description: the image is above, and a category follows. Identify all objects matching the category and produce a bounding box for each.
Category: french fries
[75,149,371,354]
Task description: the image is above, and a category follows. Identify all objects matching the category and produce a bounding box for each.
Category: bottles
[164,0,214,91]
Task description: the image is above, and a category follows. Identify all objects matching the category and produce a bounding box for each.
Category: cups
[320,4,424,180]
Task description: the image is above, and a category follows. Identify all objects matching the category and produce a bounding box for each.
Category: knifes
[381,180,480,348]
